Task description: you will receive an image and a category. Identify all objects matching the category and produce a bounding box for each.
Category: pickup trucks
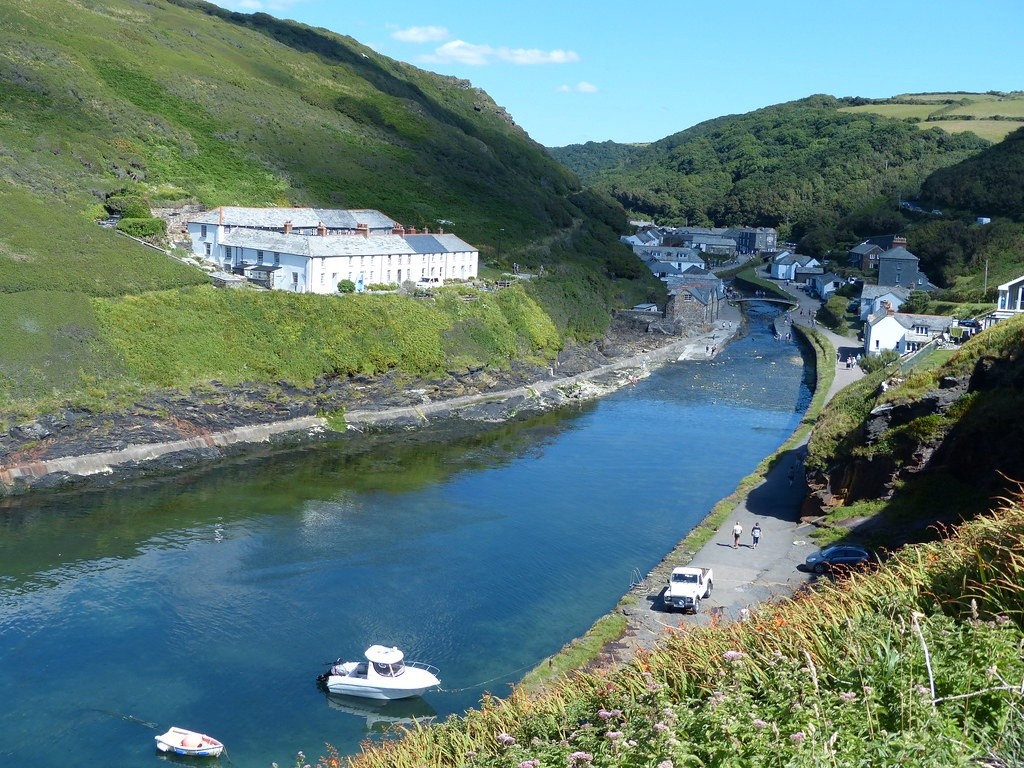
[663,565,714,613]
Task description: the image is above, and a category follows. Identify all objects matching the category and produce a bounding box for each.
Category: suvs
[806,544,874,574]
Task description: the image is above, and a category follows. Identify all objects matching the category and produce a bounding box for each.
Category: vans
[416,275,443,291]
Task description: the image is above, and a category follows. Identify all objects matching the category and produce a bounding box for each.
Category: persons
[704,306,862,371]
[732,522,742,549]
[751,523,762,549]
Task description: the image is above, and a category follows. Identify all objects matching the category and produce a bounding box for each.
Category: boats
[315,644,441,700]
[325,693,438,738]
[155,727,223,758]
[155,749,224,768]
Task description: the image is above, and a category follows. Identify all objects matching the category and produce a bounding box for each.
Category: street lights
[498,229,505,261]
